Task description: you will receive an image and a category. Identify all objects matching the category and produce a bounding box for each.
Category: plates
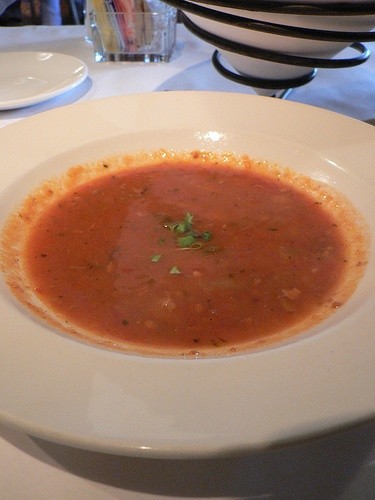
[0,91,375,457]
[0,51,88,110]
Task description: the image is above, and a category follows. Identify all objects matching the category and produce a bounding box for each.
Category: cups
[89,0,178,63]
